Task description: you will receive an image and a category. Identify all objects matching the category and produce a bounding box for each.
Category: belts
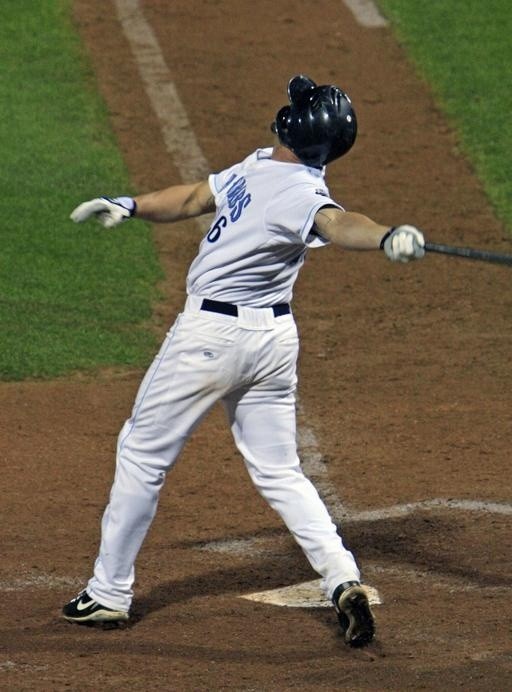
[201,299,291,319]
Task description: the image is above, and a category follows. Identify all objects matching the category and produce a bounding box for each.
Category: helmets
[276,74,357,168]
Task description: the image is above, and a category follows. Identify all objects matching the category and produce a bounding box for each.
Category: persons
[59,75,427,652]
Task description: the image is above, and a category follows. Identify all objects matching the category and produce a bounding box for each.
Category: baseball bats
[425,243,512,268]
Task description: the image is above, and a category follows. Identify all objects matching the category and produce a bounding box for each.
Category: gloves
[379,225,425,263]
[69,196,137,230]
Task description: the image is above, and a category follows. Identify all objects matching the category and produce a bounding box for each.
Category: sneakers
[330,580,376,649]
[62,592,130,622]
